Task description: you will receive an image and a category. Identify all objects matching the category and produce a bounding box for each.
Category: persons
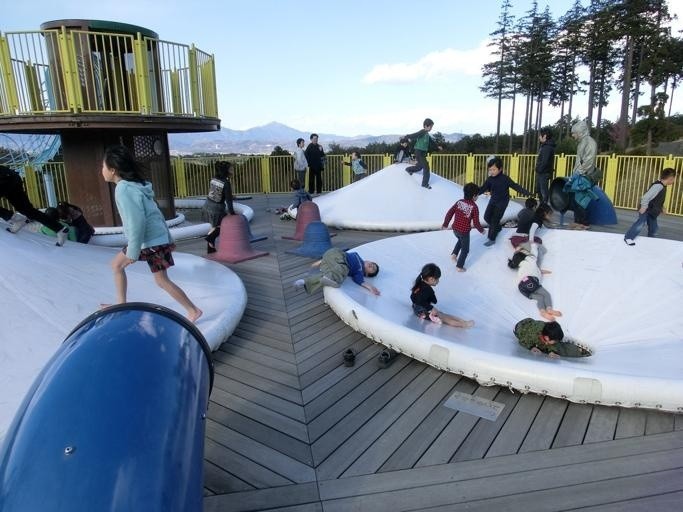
[441,183,486,271]
[339,151,369,184]
[96,144,202,325]
[508,243,561,320]
[0,165,70,247]
[293,138,308,192]
[410,262,475,329]
[292,245,381,299]
[283,179,313,221]
[53,199,94,245]
[536,130,554,202]
[566,121,597,229]
[515,317,564,357]
[479,158,529,246]
[202,160,236,255]
[625,169,676,245]
[405,119,442,189]
[512,196,550,274]
[303,133,326,197]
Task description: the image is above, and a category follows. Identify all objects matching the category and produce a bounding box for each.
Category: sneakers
[378,348,396,368]
[343,348,355,367]
